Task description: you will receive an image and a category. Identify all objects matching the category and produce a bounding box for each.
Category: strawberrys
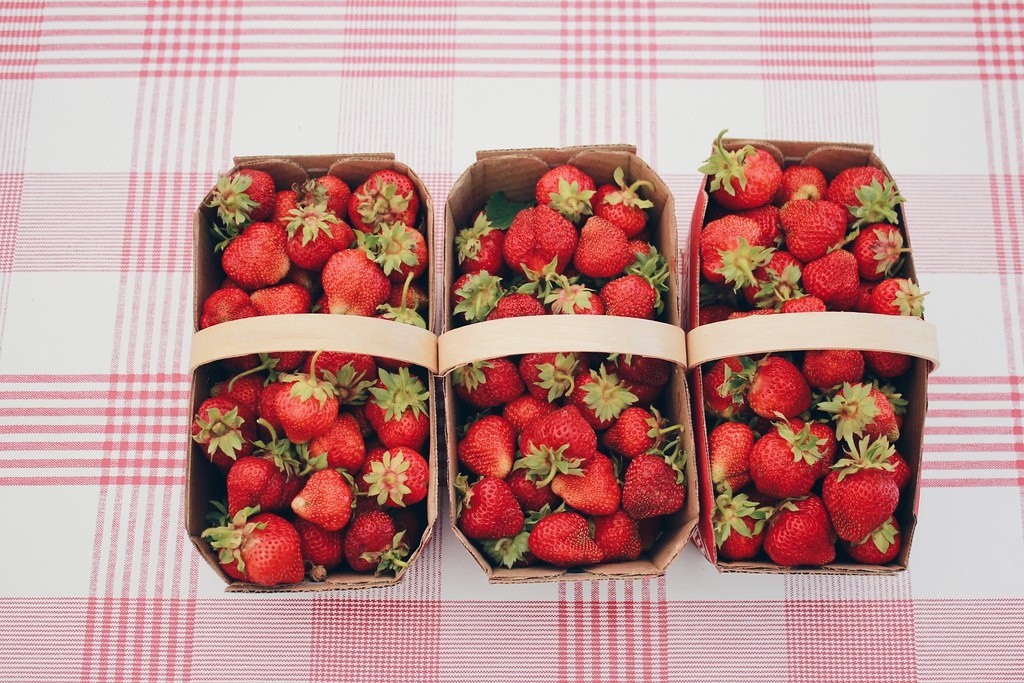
[186,168,429,590]
[449,165,690,570]
[696,131,930,571]
